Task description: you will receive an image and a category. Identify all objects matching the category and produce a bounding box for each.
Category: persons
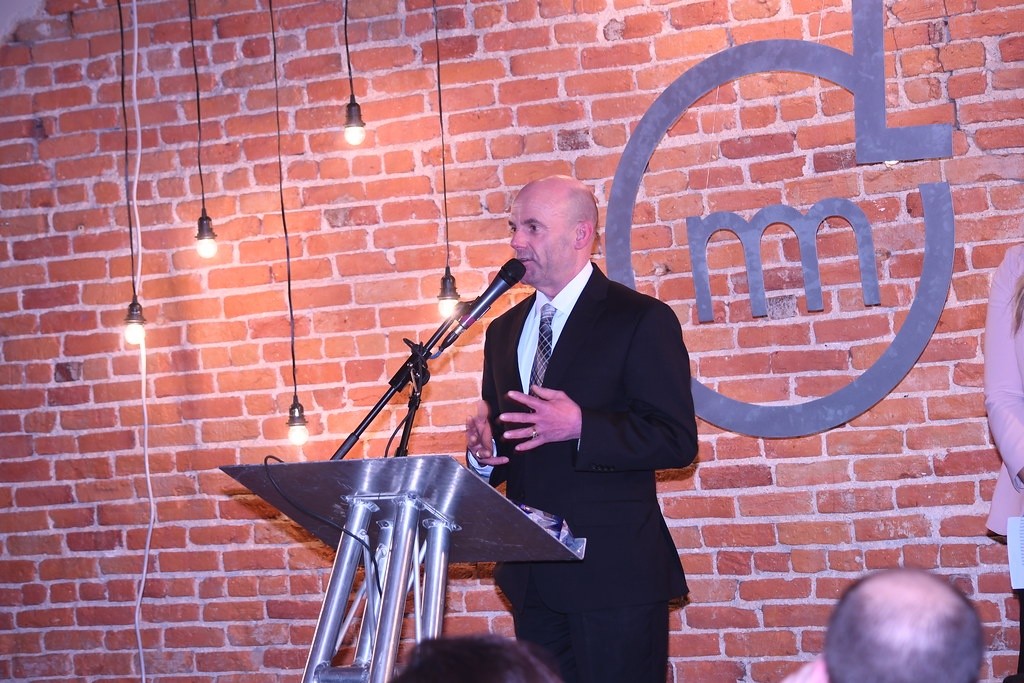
[386,632,565,683]
[982,238,1024,683]
[465,175,698,681]
[778,567,986,683]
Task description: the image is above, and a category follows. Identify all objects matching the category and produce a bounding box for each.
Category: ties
[527,303,557,415]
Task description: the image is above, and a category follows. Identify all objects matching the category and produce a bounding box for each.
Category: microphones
[442,258,527,349]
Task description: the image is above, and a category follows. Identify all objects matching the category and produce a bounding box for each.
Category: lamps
[431,1,464,320]
[112,0,148,342]
[264,0,311,446]
[186,0,222,259]
[339,0,369,148]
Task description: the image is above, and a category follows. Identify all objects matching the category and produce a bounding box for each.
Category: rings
[475,448,482,459]
[532,425,538,438]
